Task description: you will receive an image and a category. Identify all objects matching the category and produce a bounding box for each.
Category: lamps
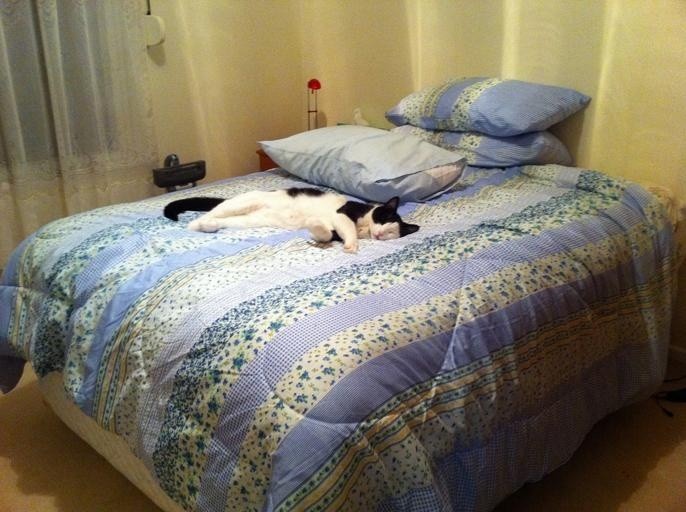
[307,79,321,130]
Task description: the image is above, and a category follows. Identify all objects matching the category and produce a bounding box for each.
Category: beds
[0,164,686,512]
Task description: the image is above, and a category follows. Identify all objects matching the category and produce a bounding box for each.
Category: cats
[163,186,420,255]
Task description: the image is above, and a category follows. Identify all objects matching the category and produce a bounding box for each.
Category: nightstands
[256,149,280,172]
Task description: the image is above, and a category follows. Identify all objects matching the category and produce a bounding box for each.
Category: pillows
[390,124,574,167]
[384,76,592,137]
[258,124,466,205]
